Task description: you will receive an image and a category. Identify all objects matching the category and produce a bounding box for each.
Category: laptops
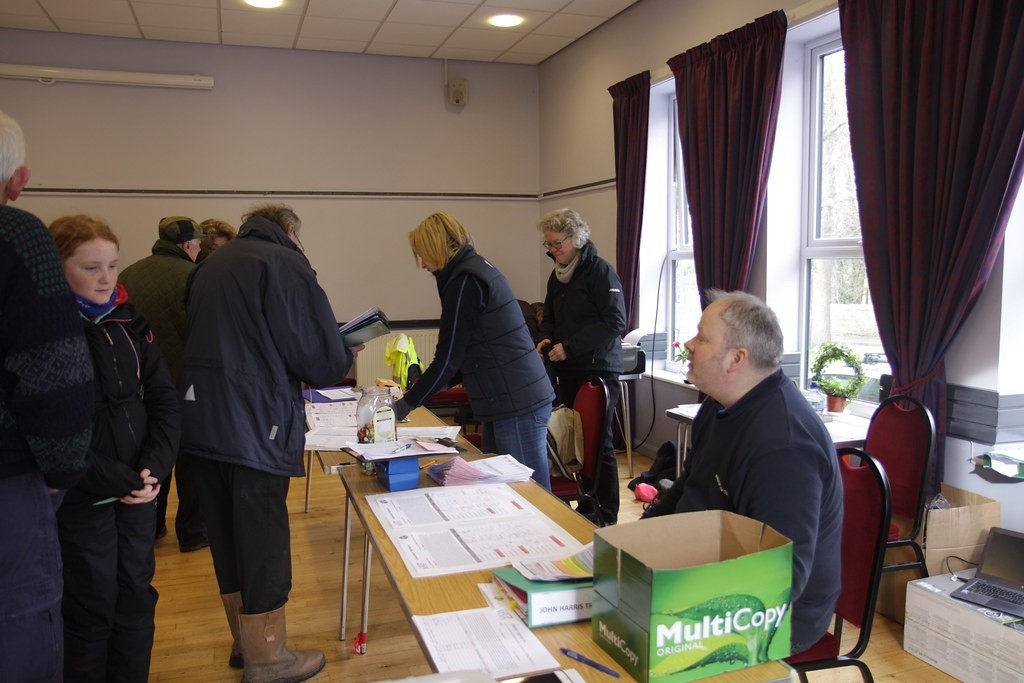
[950,527,1024,617]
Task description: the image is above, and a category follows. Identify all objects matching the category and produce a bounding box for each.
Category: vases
[827,396,846,413]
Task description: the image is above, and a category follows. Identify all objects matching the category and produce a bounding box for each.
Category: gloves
[390,395,415,423]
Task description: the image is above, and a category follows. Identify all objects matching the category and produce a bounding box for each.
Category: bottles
[357,385,398,476]
[804,381,828,423]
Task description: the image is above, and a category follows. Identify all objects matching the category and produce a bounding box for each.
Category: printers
[620,339,646,374]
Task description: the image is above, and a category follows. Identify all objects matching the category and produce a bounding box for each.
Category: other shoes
[154,524,168,543]
[178,540,211,552]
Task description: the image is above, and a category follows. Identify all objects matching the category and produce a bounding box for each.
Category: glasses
[542,235,570,250]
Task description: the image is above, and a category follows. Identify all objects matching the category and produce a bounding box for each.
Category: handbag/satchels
[545,404,585,481]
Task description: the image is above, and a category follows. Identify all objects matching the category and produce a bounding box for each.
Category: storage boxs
[590,509,794,683]
[903,567,1024,683]
[376,455,420,493]
[875,481,1000,627]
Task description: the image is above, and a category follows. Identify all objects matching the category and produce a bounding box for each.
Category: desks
[666,406,871,481]
[305,385,795,683]
[614,374,641,478]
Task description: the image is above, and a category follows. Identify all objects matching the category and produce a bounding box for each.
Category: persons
[0,108,95,683]
[180,206,366,683]
[536,209,627,528]
[116,215,209,553]
[639,288,844,656]
[392,213,556,493]
[47,215,180,683]
[195,218,236,264]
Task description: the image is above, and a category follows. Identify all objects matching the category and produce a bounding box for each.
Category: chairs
[461,375,609,527]
[834,394,935,655]
[385,332,478,434]
[778,446,892,683]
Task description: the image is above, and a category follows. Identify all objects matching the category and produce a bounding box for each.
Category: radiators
[355,328,438,384]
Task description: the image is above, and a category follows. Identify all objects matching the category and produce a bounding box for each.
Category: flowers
[810,342,867,399]
[673,340,689,362]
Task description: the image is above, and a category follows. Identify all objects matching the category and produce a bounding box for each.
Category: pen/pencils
[561,648,618,676]
[420,460,437,469]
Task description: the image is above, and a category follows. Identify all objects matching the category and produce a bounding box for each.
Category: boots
[232,604,328,683]
[219,588,252,669]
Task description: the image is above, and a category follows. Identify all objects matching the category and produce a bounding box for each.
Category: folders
[490,568,593,627]
[341,313,391,347]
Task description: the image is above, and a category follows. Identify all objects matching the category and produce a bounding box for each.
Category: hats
[158,216,209,243]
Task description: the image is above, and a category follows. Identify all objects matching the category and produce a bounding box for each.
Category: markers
[393,443,411,455]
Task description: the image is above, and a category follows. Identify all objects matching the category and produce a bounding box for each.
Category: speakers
[450,78,469,106]
